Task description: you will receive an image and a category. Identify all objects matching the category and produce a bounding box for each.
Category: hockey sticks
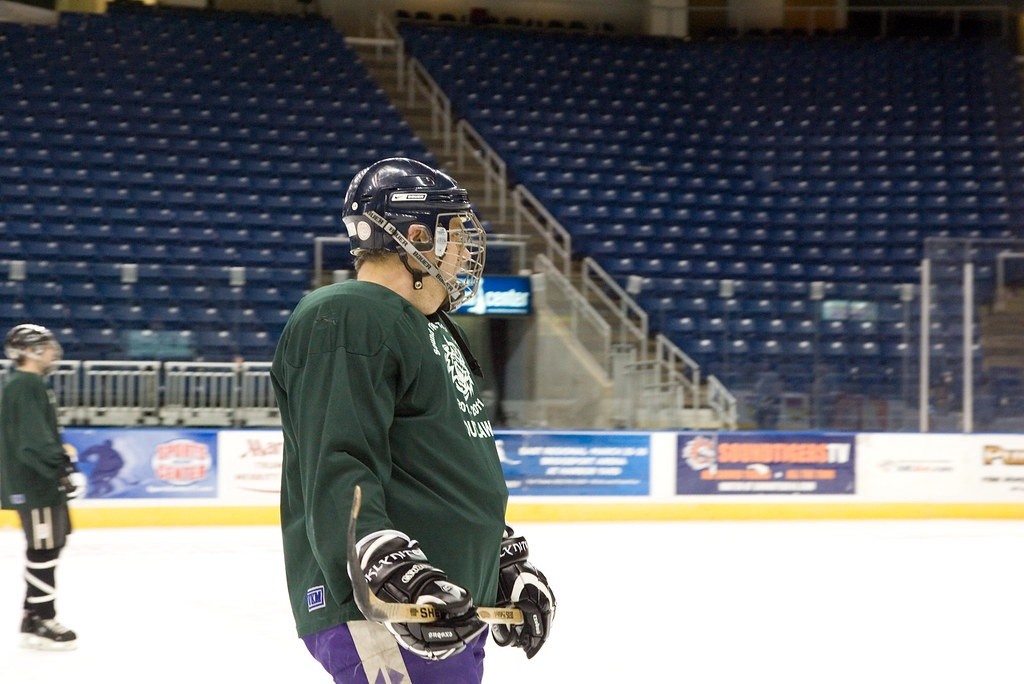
[345,483,523,626]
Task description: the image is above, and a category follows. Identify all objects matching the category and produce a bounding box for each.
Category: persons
[265,156,555,683]
[79,438,125,497]
[1,325,77,650]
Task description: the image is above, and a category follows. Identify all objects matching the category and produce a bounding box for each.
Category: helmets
[342,157,472,256]
[3,324,64,360]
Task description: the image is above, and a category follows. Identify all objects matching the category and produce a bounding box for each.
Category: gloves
[59,472,87,500]
[347,529,489,660]
[490,537,555,659]
[62,444,79,462]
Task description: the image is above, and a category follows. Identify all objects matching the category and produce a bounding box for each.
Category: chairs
[1,9,509,410]
[388,8,1024,431]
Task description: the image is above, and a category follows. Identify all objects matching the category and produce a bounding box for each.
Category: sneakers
[19,612,77,652]
